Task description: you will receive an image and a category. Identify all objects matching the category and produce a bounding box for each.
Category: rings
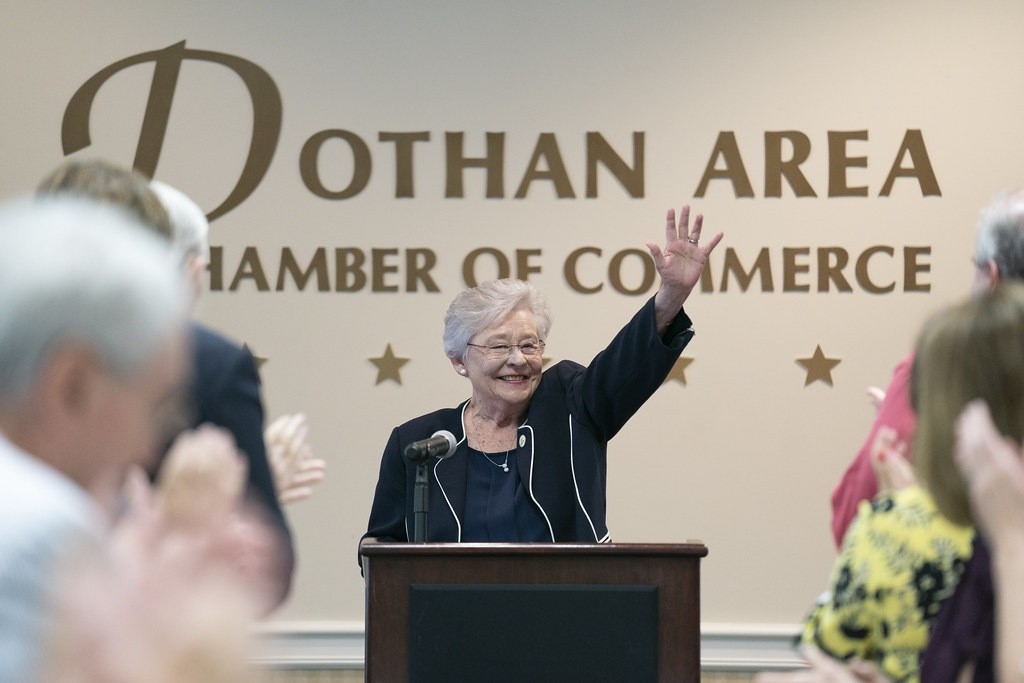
[688,237,698,243]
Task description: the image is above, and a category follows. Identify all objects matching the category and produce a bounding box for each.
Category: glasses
[467,338,546,361]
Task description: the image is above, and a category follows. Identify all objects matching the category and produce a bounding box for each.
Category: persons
[756,208,1024,683]
[0,158,326,683]
[366,205,723,544]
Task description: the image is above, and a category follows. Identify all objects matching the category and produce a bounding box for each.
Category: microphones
[405,430,457,460]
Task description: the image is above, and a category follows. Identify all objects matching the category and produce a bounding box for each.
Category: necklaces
[472,434,516,472]
